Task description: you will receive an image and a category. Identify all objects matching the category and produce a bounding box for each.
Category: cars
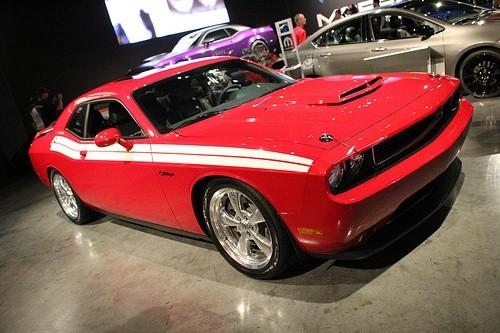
[128,24,279,76]
[285,0,500,99]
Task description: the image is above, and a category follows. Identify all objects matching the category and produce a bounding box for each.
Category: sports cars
[28,55,475,280]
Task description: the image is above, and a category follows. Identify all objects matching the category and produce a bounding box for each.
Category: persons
[30,88,62,135]
[373,0,380,8]
[335,10,344,20]
[291,13,306,50]
